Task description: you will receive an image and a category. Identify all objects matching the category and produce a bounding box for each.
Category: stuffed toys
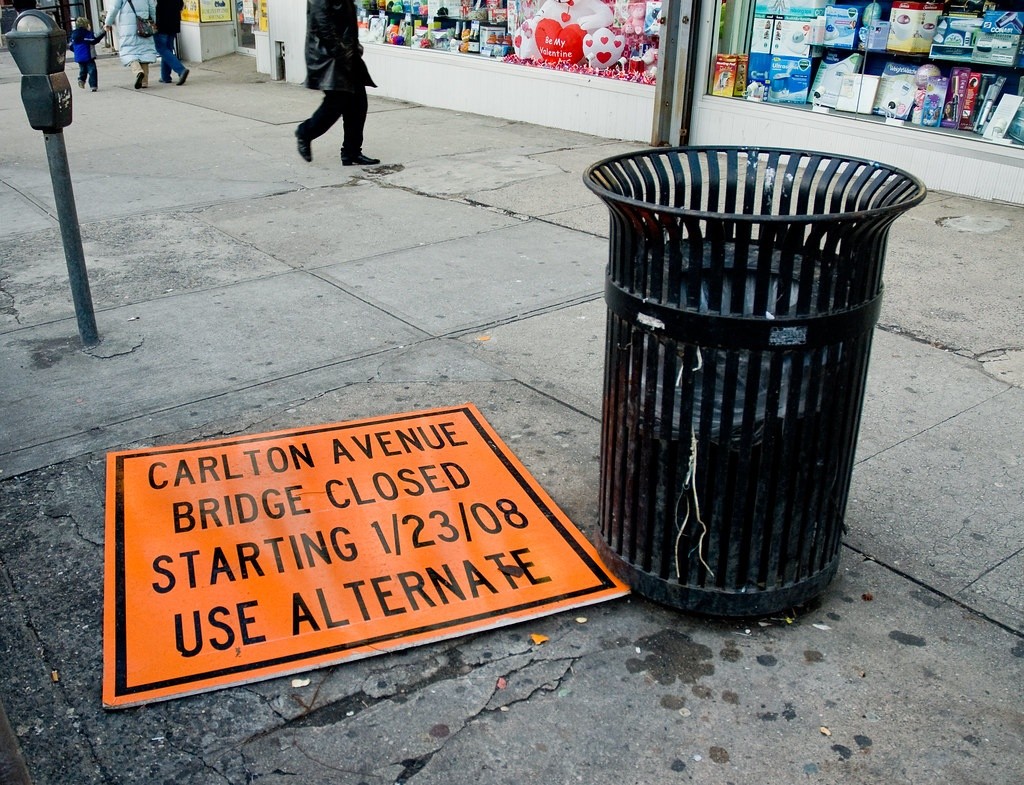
[513,0,660,79]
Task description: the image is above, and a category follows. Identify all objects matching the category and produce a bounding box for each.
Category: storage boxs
[744,0,1024,114]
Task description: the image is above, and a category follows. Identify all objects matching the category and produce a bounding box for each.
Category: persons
[153,0,190,85]
[103,0,157,88]
[70,17,108,91]
[12,0,36,14]
[295,0,383,165]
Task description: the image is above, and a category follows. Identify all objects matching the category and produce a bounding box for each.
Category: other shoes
[135,72,144,90]
[158,77,172,83]
[91,88,97,92]
[176,68,189,85]
[78,80,84,89]
[142,86,147,88]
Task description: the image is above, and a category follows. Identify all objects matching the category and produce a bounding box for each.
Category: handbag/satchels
[136,16,154,38]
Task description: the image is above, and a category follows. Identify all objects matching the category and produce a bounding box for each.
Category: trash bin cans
[576,142,931,625]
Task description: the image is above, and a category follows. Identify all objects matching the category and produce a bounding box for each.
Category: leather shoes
[342,150,380,166]
[295,125,311,162]
